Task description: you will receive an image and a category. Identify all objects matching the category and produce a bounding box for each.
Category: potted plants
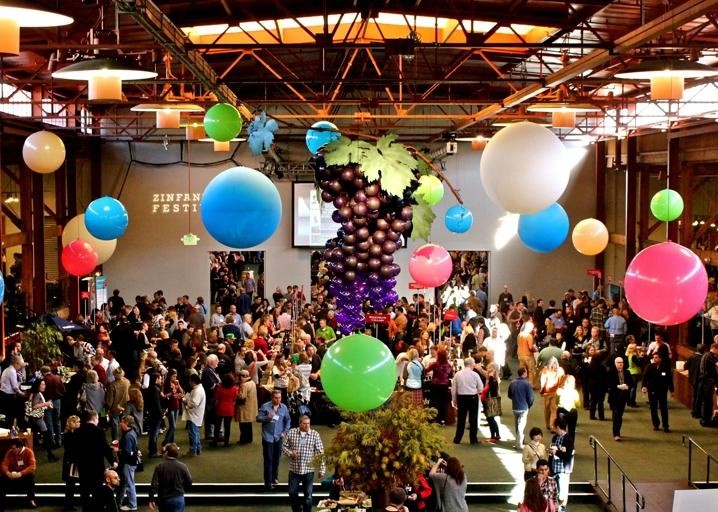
[307,387,454,512]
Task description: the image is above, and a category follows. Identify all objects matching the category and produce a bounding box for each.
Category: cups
[112,439,119,449]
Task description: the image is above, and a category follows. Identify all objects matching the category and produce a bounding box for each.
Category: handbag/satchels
[484,391,503,417]
[68,463,79,478]
[24,393,46,419]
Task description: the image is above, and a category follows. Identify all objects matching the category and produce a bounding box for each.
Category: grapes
[313,138,444,287]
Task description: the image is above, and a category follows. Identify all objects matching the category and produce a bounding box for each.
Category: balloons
[624,242,711,327]
[85,195,128,241]
[306,122,341,155]
[23,130,66,174]
[60,212,118,267]
[199,166,282,249]
[203,103,243,143]
[518,204,569,255]
[444,206,473,234]
[650,187,685,225]
[61,240,99,279]
[480,122,572,215]
[572,217,609,257]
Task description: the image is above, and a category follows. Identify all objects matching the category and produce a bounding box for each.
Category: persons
[2,251,716,511]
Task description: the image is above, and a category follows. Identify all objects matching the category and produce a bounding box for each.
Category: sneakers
[150,443,197,459]
[120,505,137,511]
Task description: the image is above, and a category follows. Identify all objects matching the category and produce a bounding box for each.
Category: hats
[225,333,237,340]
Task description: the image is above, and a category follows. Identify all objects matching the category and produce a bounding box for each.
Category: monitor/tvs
[289,181,408,251]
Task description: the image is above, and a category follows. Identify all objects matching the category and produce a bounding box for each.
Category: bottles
[11,426,18,439]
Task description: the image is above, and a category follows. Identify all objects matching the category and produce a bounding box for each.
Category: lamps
[454,133,493,152]
[50,51,159,103]
[0,0,76,58]
[526,81,602,128]
[129,95,249,154]
[613,3,718,101]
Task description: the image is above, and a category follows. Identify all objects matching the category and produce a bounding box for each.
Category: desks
[0,429,34,454]
[672,369,718,417]
[241,270,255,281]
